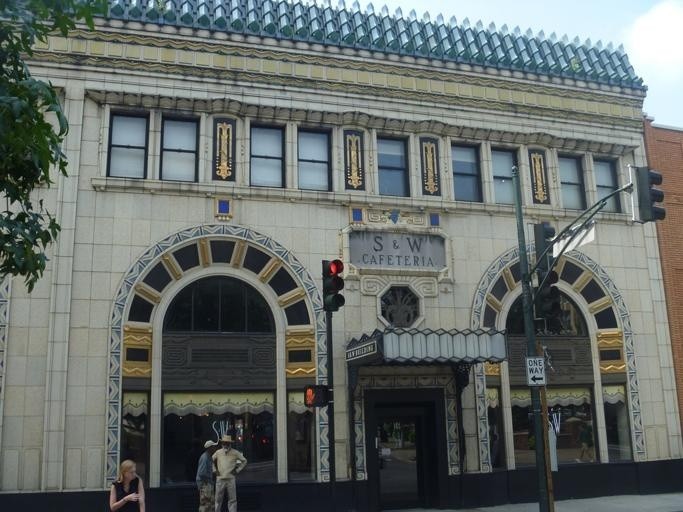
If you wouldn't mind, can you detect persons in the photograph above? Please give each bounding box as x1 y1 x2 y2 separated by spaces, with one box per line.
210 432 247 511
108 460 146 511
195 439 215 511
575 421 594 463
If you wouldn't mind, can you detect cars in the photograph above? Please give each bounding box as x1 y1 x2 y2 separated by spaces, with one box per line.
553 416 591 447
233 409 312 474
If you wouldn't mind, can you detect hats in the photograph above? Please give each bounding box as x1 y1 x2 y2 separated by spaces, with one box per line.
220 435 234 442
204 440 217 448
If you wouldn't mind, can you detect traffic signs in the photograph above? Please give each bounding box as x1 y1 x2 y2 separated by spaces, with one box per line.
524 356 547 387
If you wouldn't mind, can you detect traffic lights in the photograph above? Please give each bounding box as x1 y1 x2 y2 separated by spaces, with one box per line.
537 268 560 317
321 259 346 311
634 166 666 223
304 384 328 408
533 222 555 268
